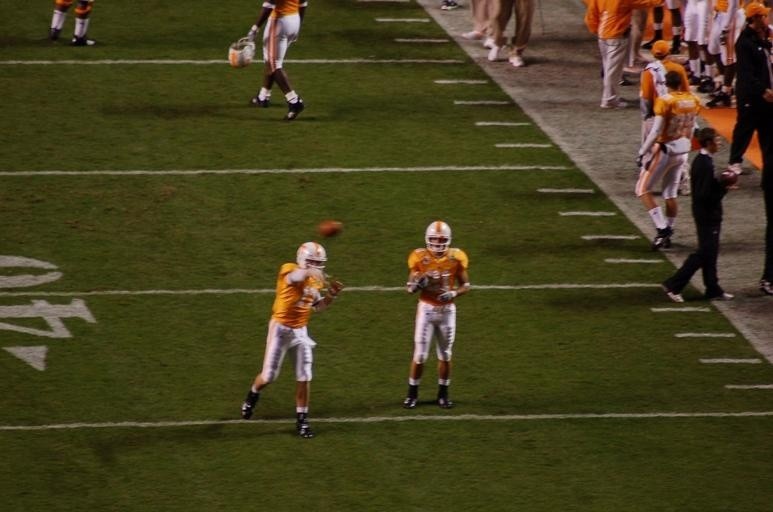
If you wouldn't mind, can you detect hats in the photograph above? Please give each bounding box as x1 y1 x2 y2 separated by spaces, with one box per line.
653 40 670 56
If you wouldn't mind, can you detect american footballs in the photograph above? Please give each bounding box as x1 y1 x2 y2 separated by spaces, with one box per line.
318 220 342 234
721 168 738 185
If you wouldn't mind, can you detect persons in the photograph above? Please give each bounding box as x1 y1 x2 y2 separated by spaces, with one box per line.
440 0 535 67
242 241 344 439
403 221 471 409
245 0 308 122
49 0 96 47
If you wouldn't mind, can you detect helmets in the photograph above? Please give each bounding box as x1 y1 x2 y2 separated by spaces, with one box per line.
425 221 451 259
229 36 256 67
296 242 328 271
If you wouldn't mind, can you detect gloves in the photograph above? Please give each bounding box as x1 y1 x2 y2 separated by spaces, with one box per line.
408 276 428 294
326 280 343 298
437 290 456 302
305 268 329 285
247 25 260 41
636 155 643 167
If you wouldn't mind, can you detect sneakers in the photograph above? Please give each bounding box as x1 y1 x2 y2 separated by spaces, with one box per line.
682 60 738 108
487 46 500 61
250 97 270 107
600 102 629 108
463 30 487 39
296 419 315 438
617 79 633 87
642 39 656 49
442 1 458 10
728 163 743 175
759 279 773 295
483 33 496 47
71 35 95 45
284 96 305 120
651 226 673 250
708 293 734 300
49 28 60 40
662 284 684 303
403 393 418 409
241 400 259 419
680 189 691 195
671 44 681 55
437 397 453 408
508 51 525 67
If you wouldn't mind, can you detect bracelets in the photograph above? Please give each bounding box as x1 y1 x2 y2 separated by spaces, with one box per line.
326 289 337 299
450 289 457 298
251 24 260 33
